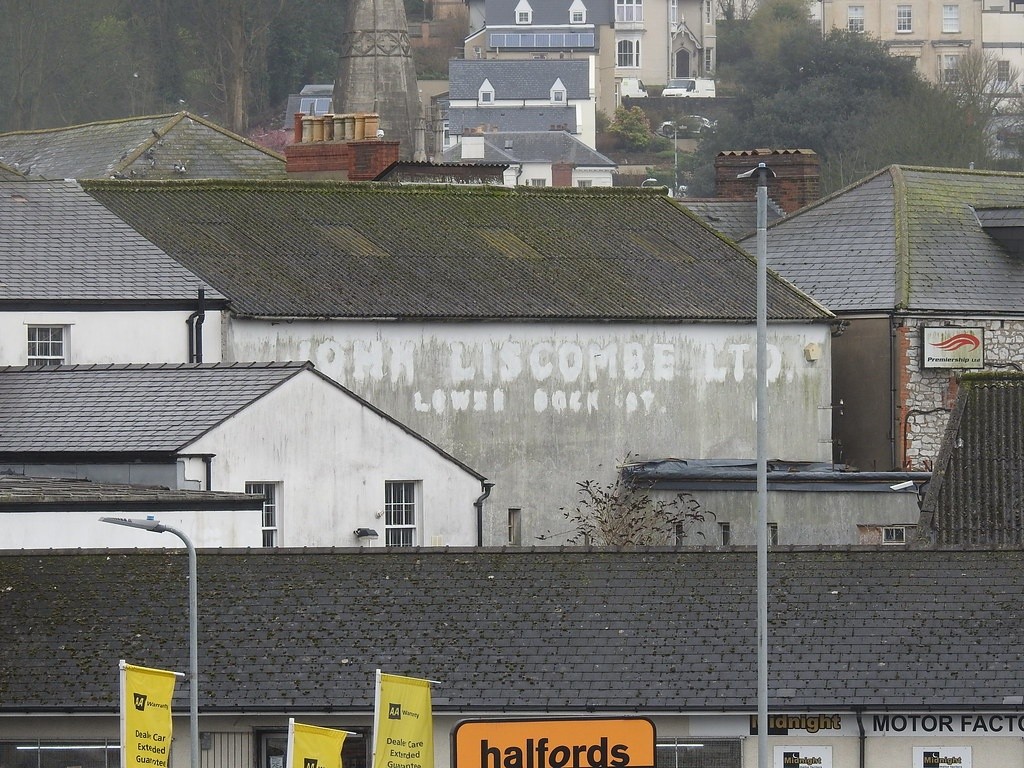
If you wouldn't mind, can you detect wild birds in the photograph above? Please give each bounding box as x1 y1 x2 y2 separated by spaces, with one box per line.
108 127 187 180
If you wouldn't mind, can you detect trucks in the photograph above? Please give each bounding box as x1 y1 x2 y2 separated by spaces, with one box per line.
616 77 649 98
659 76 715 99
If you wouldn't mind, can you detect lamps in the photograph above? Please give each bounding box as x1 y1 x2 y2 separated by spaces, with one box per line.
354 528 378 540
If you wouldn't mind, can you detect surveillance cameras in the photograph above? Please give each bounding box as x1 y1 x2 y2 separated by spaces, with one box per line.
890 481 914 491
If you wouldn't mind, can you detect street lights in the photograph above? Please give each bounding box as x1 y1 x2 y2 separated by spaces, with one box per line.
736 162 779 767
98 515 201 768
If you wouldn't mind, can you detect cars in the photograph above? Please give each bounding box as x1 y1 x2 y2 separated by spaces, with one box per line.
662 114 716 139
